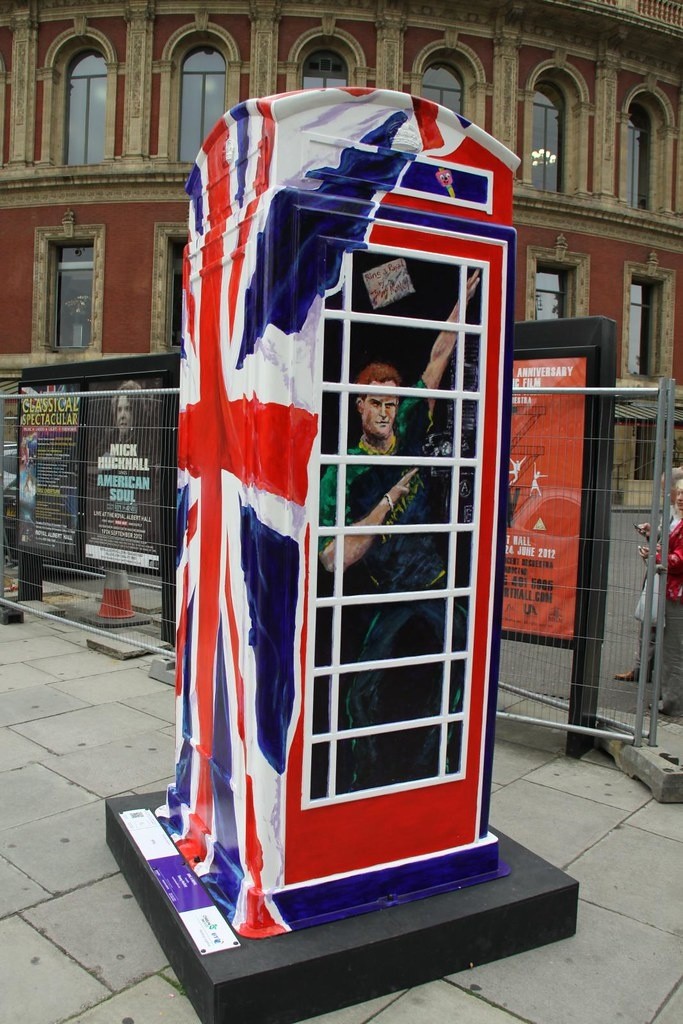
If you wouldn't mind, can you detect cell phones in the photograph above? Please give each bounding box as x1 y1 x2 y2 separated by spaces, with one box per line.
633 524 646 534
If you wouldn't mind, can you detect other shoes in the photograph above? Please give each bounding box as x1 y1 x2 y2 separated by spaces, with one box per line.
615 670 639 681
648 700 663 712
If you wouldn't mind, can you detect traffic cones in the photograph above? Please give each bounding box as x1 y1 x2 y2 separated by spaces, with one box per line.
96 567 136 618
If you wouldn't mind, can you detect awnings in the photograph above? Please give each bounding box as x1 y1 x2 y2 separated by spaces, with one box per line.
614 402 683 423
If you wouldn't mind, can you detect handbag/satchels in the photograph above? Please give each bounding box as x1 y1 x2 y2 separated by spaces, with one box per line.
633 573 666 627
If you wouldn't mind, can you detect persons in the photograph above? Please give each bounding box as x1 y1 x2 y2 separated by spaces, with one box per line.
639 480 683 718
615 468 683 683
88 378 156 457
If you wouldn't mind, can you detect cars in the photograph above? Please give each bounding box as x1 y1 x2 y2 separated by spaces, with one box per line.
3 444 19 559
